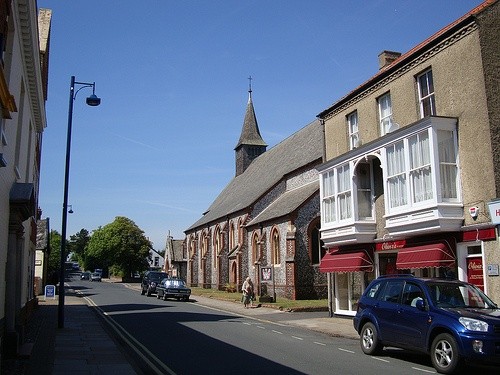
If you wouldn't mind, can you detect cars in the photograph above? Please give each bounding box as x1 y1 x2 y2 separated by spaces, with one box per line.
155 278 191 300
72 262 79 271
79 269 101 282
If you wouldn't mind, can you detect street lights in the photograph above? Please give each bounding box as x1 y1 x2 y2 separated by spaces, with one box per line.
58 75 102 330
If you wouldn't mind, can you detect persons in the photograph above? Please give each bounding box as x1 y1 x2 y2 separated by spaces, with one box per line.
241 277 255 308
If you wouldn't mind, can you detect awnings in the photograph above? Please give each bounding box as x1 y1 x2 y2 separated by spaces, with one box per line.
319 247 375 273
396 235 456 270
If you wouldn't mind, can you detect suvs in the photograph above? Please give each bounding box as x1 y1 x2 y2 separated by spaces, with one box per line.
141 271 168 297
353 273 500 373
